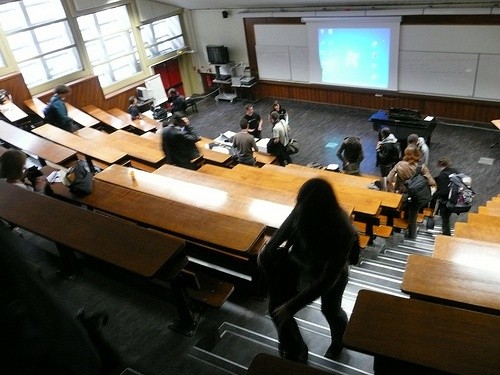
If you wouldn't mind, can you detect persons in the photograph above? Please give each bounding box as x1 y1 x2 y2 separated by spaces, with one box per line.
271 111 292 168
128 96 143 120
161 112 201 172
43 84 72 132
243 104 263 139
0 225 101 375
386 132 460 240
376 127 400 177
257 177 360 365
336 136 365 171
0 148 48 196
168 88 188 117
233 119 259 167
269 100 290 132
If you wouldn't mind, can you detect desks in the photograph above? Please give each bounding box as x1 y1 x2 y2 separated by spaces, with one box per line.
137 98 155 113
0 99 500 375
231 82 260 103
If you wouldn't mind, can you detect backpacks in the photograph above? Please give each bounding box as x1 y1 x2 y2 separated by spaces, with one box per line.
43 98 63 127
404 163 432 206
438 172 473 208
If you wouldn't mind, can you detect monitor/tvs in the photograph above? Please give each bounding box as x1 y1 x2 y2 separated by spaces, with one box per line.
207 45 229 64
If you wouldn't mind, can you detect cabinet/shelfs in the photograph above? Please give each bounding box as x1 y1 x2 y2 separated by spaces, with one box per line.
209 61 237 102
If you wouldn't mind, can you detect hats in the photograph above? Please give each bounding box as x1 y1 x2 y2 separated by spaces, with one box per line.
55 85 69 94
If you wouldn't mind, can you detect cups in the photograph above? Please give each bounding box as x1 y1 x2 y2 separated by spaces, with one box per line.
129 170 135 180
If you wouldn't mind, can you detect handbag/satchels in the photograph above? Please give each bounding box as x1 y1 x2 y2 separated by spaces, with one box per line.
153 108 167 120
286 138 299 155
66 164 93 199
257 245 302 293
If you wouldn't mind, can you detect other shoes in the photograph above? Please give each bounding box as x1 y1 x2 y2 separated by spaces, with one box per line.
323 339 344 361
405 233 414 240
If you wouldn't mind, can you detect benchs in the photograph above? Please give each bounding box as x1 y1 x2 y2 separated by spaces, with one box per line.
130 159 156 173
352 221 393 237
379 215 410 229
76 153 109 170
184 272 235 309
